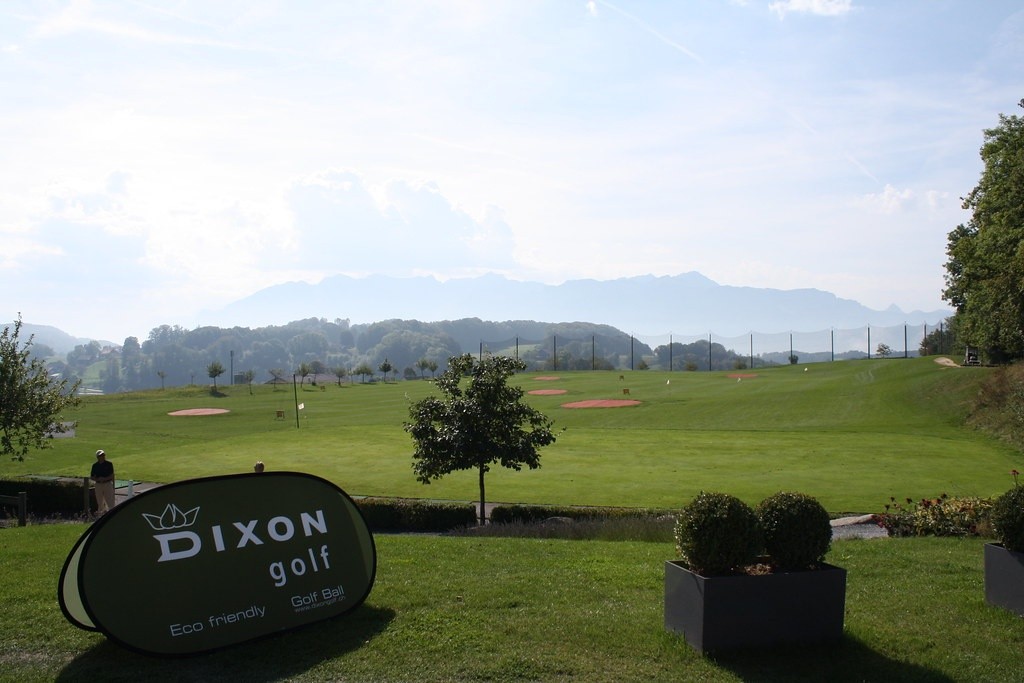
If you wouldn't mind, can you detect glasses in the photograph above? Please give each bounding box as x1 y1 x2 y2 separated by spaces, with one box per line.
97 454 105 458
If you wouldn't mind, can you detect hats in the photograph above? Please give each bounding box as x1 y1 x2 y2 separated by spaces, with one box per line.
96 450 105 457
254 463 264 470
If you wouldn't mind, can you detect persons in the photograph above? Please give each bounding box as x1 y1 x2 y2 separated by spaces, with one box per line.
253 462 264 472
91 450 115 517
